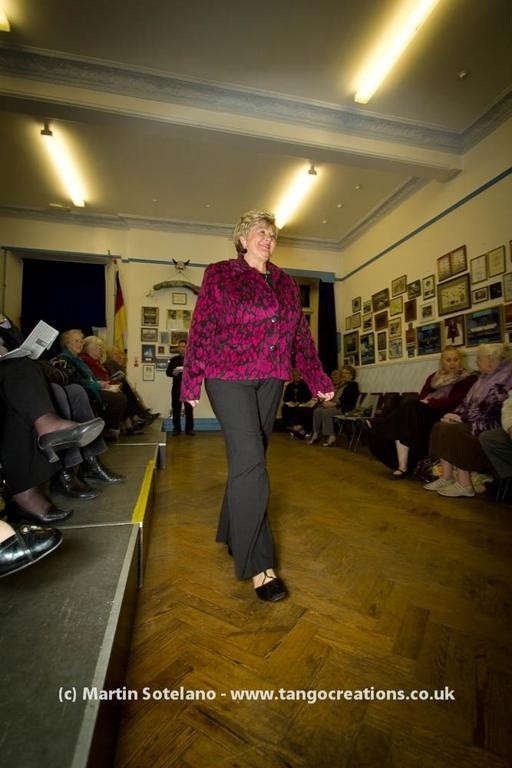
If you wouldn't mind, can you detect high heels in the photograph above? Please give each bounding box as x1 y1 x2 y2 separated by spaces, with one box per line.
35 417 105 464
6 496 74 524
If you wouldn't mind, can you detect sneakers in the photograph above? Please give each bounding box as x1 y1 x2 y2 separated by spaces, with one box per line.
305 436 321 445
421 476 457 491
322 439 338 447
435 479 477 497
387 467 410 480
250 568 290 602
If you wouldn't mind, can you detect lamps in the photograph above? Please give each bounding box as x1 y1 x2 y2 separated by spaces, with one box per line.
39 118 88 209
274 161 318 232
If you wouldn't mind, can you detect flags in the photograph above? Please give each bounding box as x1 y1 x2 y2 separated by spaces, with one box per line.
113 263 127 352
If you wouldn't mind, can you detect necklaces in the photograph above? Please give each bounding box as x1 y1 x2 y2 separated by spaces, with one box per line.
263 270 269 286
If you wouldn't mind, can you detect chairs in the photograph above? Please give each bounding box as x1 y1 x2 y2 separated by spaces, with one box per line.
331 392 421 457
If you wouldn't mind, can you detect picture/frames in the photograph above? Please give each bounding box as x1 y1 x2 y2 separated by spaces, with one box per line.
141 293 194 381
343 239 512 370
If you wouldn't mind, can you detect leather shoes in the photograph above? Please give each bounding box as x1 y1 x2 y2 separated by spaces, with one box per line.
47 469 102 502
121 409 197 438
76 455 129 486
0 522 63 579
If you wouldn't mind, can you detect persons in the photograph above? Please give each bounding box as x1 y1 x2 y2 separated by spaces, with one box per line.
166 340 195 436
180 212 335 602
479 389 512 502
281 365 358 447
424 343 512 497
361 346 478 479
